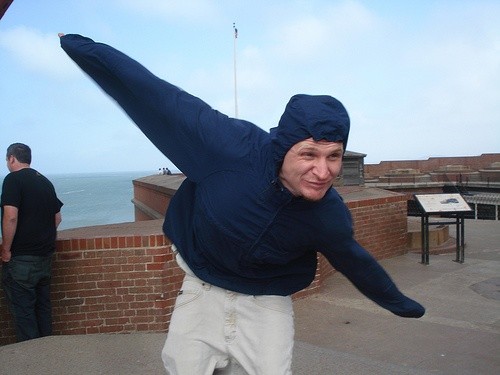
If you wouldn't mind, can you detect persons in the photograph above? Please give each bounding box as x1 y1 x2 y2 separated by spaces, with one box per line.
158 168 162 175
165 168 171 175
0 142 64 342
162 168 167 175
58 31 425 374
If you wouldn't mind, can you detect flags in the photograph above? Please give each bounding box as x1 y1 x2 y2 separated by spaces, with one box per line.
233 27 238 39
